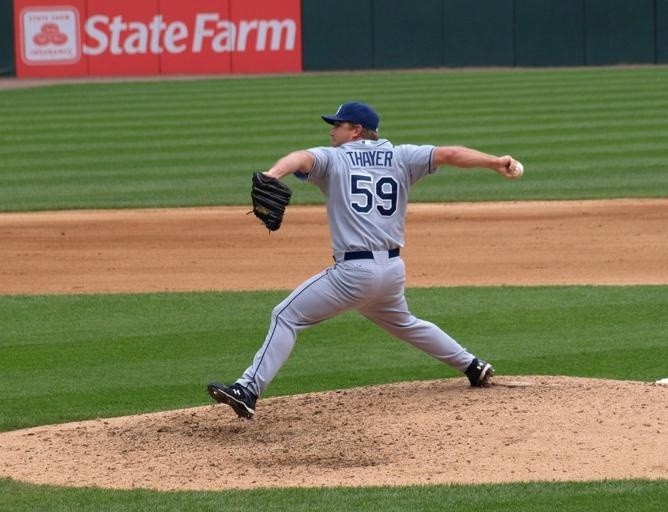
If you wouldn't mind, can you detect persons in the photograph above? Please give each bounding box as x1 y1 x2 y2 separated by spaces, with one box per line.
208 99 523 419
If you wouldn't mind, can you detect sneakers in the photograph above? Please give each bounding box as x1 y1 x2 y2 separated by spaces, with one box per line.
465 356 496 388
204 381 258 420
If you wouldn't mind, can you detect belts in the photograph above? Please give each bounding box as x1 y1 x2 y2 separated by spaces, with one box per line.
332 248 401 262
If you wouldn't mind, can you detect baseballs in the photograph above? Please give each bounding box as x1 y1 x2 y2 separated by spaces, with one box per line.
510 161 524 180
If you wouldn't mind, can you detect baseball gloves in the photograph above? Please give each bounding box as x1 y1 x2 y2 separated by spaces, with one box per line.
245 172 292 235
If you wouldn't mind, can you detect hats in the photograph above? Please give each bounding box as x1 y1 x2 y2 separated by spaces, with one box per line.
321 100 382 132
